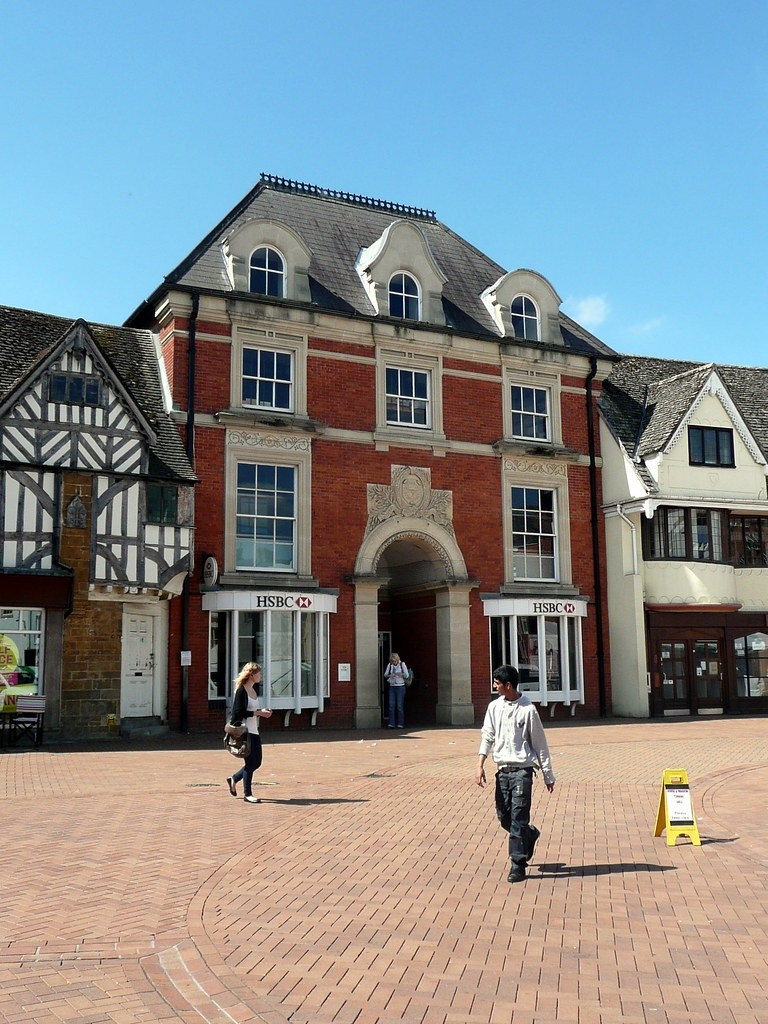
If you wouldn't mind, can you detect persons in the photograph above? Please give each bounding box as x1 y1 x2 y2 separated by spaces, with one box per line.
475 664 556 882
384 652 411 731
222 661 273 803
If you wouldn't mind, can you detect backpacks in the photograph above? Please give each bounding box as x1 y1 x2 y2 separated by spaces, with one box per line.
401 661 413 687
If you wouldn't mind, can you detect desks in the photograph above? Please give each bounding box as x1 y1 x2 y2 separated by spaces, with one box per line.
1 712 44 748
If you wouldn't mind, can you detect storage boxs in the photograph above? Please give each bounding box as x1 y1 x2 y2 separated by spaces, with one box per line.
3 695 45 713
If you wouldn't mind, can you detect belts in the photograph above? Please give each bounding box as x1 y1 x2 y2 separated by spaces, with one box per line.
502 767 520 773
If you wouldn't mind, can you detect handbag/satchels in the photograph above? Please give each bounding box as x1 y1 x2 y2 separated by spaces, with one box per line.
223 720 251 758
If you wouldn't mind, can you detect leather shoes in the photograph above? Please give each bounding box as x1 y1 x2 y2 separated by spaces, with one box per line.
507 859 526 882
526 825 540 861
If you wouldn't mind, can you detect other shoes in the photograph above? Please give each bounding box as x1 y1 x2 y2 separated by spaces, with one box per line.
388 724 404 729
244 795 261 803
227 776 237 796
